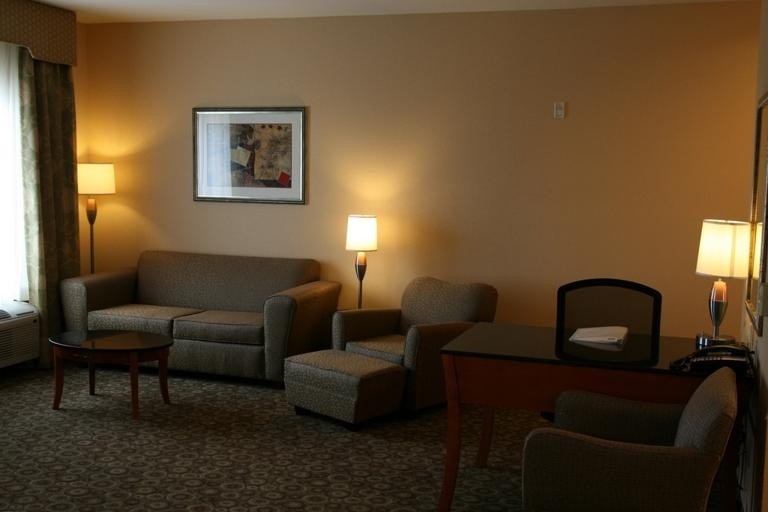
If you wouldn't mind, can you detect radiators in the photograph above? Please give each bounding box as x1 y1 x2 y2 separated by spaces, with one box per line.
0 312 39 368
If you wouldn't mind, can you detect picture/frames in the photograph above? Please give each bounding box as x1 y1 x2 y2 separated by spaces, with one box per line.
192 106 306 203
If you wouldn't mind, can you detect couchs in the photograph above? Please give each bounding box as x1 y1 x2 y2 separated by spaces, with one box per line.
522 367 738 512
59 250 341 424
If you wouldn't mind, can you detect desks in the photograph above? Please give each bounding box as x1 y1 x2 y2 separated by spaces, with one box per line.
437 322 760 512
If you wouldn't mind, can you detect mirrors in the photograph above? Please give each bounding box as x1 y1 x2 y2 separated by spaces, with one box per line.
744 88 768 337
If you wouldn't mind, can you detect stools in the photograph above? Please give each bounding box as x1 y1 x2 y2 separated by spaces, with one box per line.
285 349 407 433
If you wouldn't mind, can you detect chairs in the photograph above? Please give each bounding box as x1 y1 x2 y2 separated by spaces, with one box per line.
540 277 659 425
331 277 498 414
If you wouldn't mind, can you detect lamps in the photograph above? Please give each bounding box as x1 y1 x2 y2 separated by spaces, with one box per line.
696 219 755 350
76 164 118 275
345 214 379 309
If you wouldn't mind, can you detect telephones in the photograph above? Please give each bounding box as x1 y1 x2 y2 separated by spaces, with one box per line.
675 343 749 372
